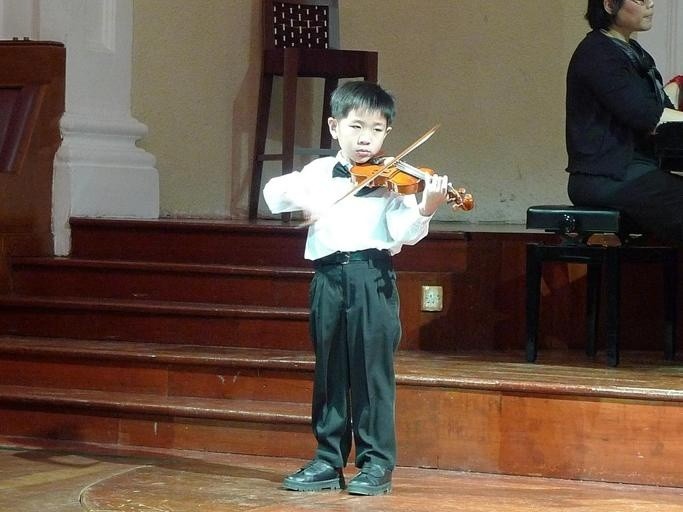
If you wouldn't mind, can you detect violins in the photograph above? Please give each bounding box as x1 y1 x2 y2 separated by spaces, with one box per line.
349 154 475 212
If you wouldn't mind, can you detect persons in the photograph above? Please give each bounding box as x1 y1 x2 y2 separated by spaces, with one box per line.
260 79 449 496
564 0 683 249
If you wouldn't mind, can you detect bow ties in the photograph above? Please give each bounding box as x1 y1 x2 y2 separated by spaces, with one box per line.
333 161 351 178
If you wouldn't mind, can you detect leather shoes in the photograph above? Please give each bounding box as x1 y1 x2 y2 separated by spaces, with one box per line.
348 465 392 496
283 459 345 492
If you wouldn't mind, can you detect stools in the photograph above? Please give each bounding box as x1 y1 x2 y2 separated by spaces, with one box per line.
518 203 679 370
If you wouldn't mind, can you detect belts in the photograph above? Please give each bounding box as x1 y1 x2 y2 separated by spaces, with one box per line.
314 250 388 265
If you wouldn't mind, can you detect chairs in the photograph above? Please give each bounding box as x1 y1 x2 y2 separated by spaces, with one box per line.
246 0 378 224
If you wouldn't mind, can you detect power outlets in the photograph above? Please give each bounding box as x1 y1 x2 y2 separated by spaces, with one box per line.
419 284 443 312
